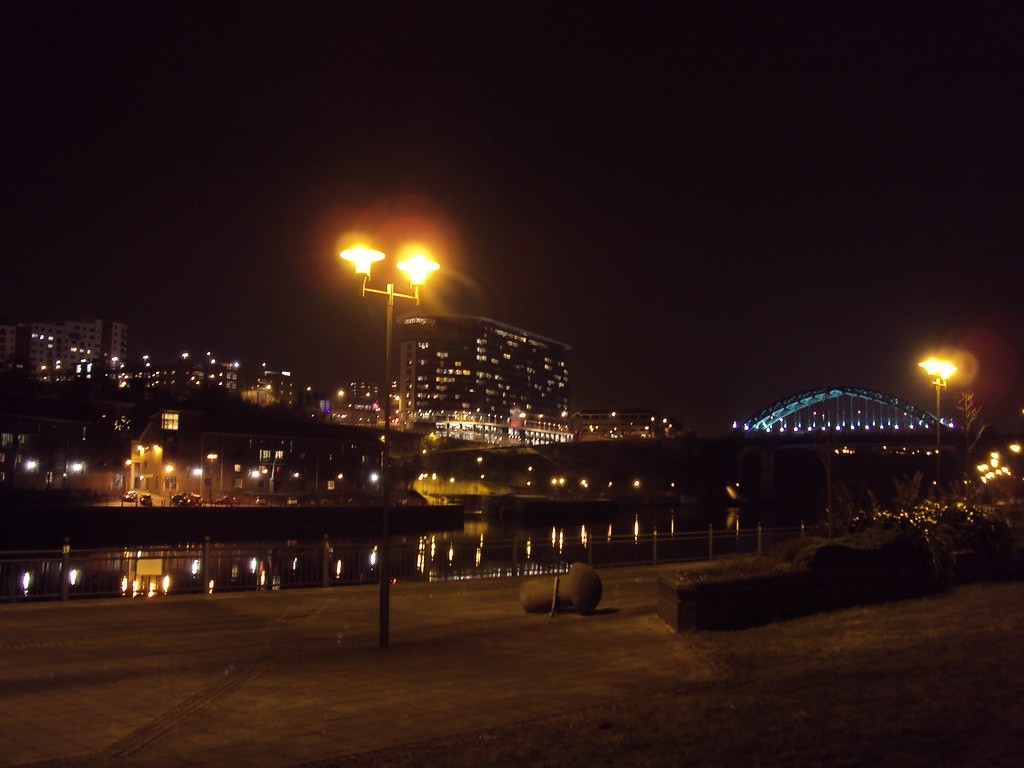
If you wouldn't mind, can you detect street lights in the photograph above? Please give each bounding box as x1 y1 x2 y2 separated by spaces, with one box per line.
207 453 218 505
338 241 441 650
919 354 959 504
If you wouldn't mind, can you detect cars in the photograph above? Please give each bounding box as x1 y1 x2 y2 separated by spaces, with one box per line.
140 493 152 506
287 496 298 507
124 490 138 502
171 491 204 506
215 495 236 506
